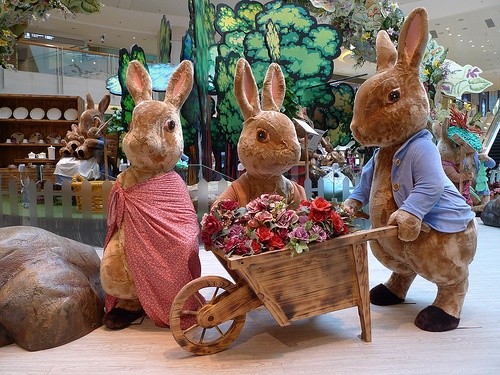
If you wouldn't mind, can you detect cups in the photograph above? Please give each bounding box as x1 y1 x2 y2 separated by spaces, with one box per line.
37 152 46 158
28 152 35 158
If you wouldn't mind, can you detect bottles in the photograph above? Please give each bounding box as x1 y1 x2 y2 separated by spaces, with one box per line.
48 145 55 160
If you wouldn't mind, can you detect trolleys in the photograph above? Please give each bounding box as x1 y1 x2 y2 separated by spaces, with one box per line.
170 207 431 356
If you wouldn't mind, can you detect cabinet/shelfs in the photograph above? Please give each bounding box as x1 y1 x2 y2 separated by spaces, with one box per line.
0 94 84 191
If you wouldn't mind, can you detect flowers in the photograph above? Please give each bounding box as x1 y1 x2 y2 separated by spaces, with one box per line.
198 192 353 254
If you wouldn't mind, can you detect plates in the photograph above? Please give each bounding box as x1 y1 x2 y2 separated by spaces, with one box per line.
46 133 61 144
0 107 12 119
29 133 43 144
13 107 28 119
30 108 46 119
10 132 24 144
64 109 78 120
47 108 62 120
